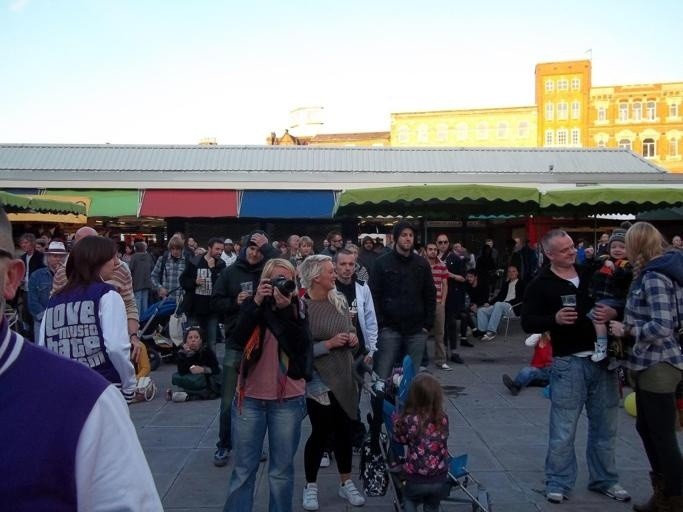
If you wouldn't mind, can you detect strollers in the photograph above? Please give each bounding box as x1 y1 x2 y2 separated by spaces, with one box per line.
349 354 496 511
139 289 180 370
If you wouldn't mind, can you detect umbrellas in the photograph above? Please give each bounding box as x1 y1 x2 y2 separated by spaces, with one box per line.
540 187 683 256
331 186 541 247
0 190 87 215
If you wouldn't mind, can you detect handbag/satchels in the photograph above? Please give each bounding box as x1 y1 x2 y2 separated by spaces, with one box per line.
169 312 187 346
136 377 155 402
172 371 208 391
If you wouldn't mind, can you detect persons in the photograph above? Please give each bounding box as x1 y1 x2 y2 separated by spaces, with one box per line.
671 236 682 248
610 223 682 511
583 221 635 370
1 204 556 512
520 227 631 505
575 232 610 261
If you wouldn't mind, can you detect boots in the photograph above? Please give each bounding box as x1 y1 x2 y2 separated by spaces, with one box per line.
633 471 683 511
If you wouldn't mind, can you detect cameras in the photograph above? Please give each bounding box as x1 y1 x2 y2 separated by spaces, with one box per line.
268 274 296 297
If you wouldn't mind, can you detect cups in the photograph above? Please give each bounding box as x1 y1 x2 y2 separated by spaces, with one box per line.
240 281 254 296
560 294 577 322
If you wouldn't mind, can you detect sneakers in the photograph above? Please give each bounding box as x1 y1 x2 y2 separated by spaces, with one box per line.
546 493 565 504
172 391 188 402
600 484 631 502
503 374 520 396
213 447 365 510
591 343 608 363
435 329 497 371
607 357 623 371
362 430 389 497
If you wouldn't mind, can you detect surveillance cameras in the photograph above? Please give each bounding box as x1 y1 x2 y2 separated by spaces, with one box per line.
549 165 553 171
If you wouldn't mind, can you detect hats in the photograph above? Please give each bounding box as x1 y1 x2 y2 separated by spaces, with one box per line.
607 221 632 254
392 219 416 240
43 241 70 255
246 231 270 247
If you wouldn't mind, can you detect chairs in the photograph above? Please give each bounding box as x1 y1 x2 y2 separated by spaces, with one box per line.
503 303 521 342
